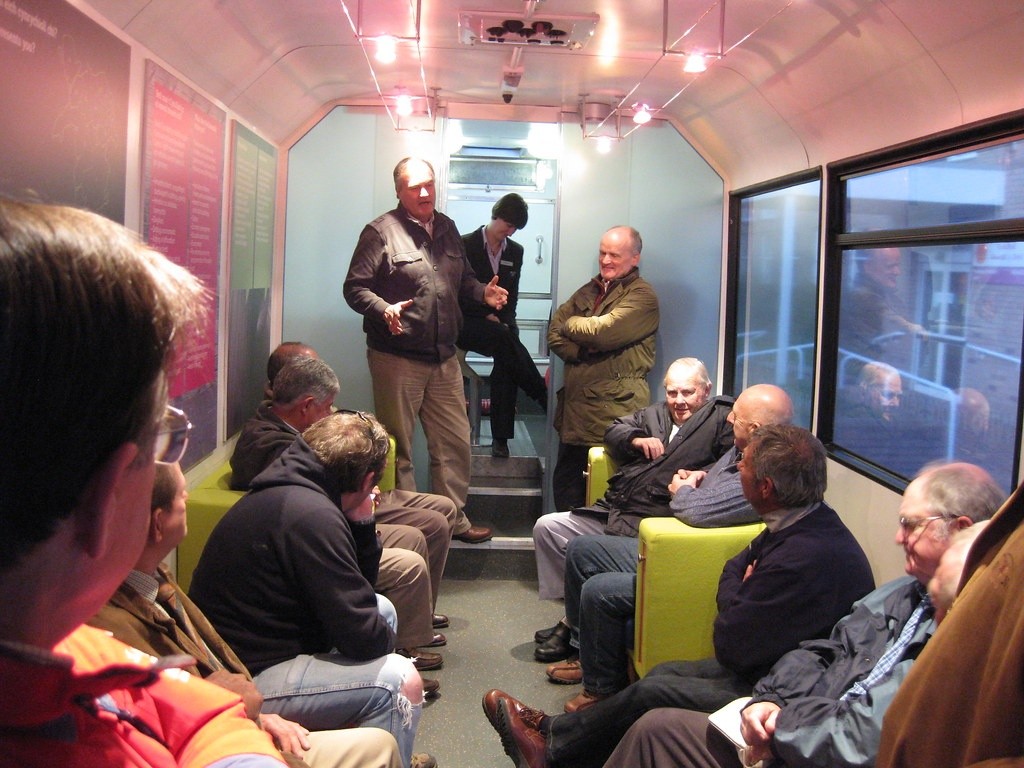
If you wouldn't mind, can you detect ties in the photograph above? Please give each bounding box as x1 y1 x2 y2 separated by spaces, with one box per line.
156 577 225 671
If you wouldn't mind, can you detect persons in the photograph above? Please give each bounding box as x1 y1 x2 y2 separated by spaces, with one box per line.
548 225 660 512
604 459 1023 767
835 246 996 491
545 383 794 714
482 423 876 768
187 407 437 768
84 462 405 768
262 340 457 646
0 198 285 768
458 193 548 458
343 157 493 544
228 353 445 698
533 356 736 663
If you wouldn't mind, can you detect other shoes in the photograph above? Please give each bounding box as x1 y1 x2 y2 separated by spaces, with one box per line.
491 439 509 458
538 380 549 415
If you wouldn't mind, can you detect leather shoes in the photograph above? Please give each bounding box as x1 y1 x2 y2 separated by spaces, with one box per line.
563 688 604 713
482 688 546 734
534 627 554 643
395 647 444 670
496 696 551 768
432 613 450 628
425 633 446 647
450 525 493 544
546 659 584 684
533 615 577 662
422 678 440 698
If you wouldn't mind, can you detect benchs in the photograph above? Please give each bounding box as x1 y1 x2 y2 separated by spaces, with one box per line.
176 434 396 597
583 446 767 680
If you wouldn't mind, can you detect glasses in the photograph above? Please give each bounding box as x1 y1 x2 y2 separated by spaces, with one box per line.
334 409 376 444
90 406 195 465
900 514 976 537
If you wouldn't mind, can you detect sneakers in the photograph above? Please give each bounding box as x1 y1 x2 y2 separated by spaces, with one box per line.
409 752 437 768
